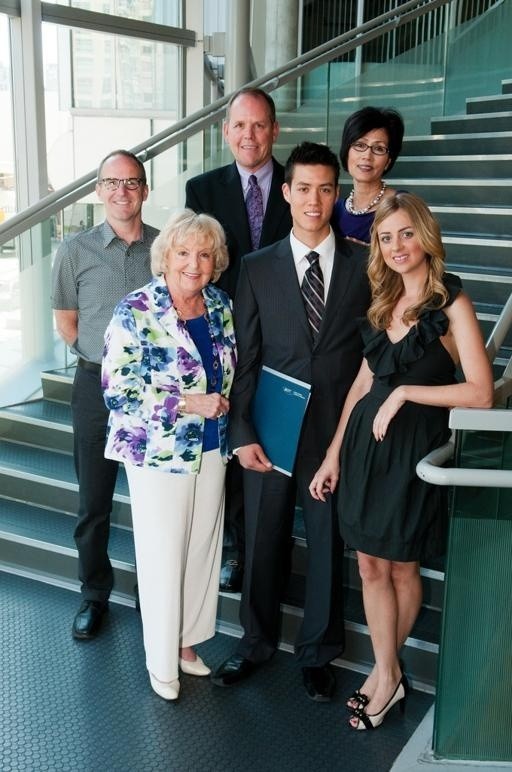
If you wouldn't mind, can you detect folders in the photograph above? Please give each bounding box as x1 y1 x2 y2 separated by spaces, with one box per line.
249 365 313 479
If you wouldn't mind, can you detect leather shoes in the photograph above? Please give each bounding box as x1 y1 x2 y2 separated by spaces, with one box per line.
220 559 243 593
149 673 180 701
212 653 256 687
303 665 340 703
178 654 212 675
72 600 108 640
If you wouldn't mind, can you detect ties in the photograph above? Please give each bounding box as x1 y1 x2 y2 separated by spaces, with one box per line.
300 251 324 347
245 175 264 250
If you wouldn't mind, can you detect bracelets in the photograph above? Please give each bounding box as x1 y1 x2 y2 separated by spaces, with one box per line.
176 394 186 412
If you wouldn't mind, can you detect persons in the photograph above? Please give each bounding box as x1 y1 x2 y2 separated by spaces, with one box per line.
210 142 375 705
309 190 493 731
331 107 405 244
50 151 163 640
184 90 294 595
101 208 239 700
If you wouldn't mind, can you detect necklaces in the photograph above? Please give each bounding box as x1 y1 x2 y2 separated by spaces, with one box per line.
345 180 386 215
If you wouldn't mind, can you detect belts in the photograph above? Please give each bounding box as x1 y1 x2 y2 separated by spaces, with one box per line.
78 357 101 374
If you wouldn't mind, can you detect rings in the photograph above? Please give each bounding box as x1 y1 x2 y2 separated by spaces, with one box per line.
218 412 224 419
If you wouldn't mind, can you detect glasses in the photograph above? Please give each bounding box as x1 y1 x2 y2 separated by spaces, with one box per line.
352 141 389 155
98 178 144 191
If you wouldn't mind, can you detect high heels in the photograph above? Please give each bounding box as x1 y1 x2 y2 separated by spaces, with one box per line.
348 675 410 730
347 659 404 711
